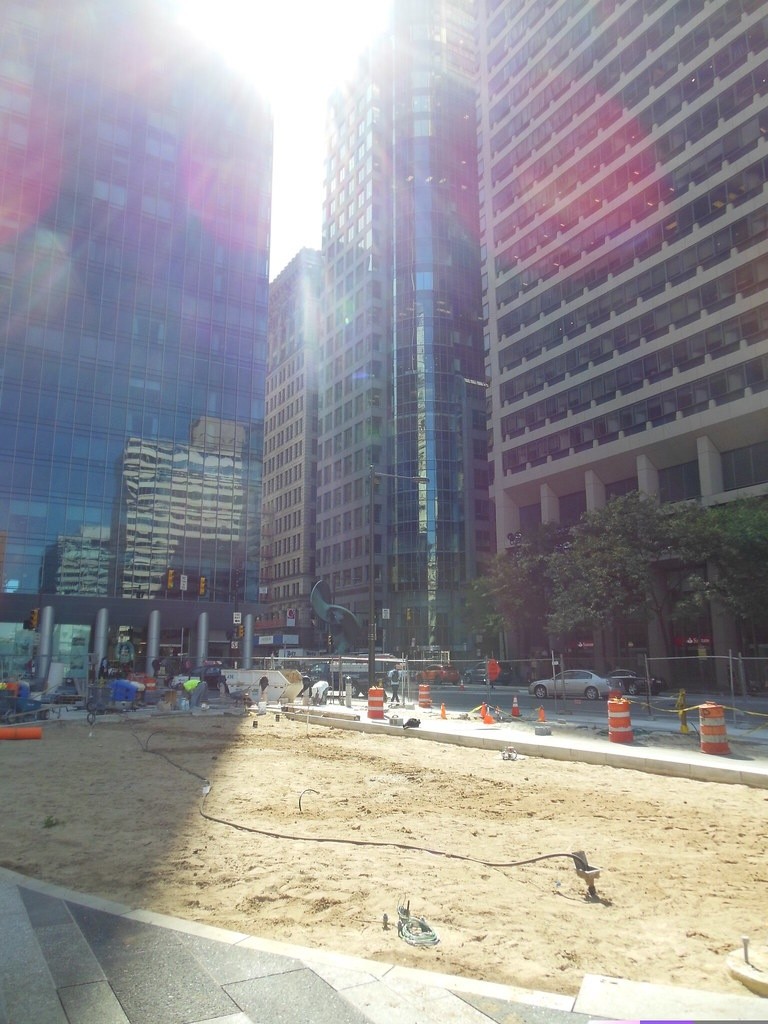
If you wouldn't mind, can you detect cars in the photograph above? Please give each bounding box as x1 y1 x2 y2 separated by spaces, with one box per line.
163 660 234 691
465 660 514 686
603 667 668 695
414 662 466 686
527 668 626 701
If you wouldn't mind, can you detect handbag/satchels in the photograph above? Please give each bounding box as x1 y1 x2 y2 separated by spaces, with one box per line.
403 718 421 730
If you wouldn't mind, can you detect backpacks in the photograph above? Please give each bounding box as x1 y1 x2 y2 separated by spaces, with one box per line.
392 670 400 682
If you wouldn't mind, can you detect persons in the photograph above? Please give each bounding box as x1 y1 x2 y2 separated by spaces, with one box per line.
388 665 400 706
101 656 108 679
152 658 160 678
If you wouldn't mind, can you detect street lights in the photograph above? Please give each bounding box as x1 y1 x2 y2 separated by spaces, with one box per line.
369 463 429 690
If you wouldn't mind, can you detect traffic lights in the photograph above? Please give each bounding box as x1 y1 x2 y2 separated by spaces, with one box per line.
30 608 38 626
199 576 207 596
328 635 333 645
238 625 245 638
168 569 175 589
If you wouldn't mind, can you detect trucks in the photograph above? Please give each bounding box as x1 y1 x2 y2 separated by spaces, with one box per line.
304 652 400 700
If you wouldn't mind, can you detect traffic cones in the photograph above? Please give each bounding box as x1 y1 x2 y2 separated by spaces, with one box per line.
456 679 467 693
483 706 495 724
509 693 523 718
479 701 487 718
535 705 549 723
440 702 449 720
